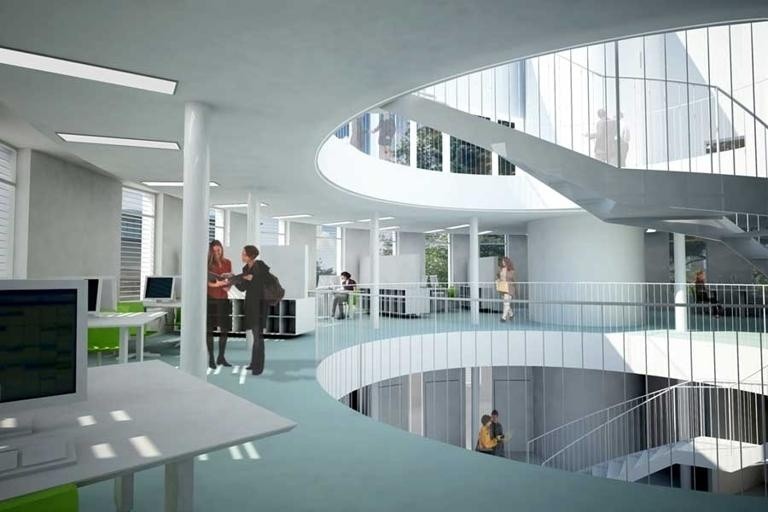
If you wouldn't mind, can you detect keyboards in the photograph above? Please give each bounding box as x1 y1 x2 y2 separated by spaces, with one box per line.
0 433 79 479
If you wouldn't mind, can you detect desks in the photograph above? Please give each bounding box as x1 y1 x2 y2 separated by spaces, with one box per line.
707 288 759 318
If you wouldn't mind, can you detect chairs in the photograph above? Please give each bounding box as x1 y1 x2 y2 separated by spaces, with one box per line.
343 286 360 320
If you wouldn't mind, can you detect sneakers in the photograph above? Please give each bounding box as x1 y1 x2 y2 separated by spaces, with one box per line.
336 314 346 319
209 359 262 375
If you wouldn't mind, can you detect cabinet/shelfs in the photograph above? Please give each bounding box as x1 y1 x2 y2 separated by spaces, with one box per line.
359 287 430 319
459 286 493 312
174 297 316 339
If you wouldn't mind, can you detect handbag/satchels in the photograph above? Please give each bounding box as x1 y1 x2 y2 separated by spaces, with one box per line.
495 280 509 293
262 273 284 307
475 448 497 456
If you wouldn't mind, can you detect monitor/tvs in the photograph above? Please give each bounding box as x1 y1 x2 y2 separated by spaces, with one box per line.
318 275 342 289
100 276 117 311
82 277 103 315
141 275 176 303
0 278 89 438
169 276 181 299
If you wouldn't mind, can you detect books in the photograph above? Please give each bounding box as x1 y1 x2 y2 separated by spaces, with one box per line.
208 270 244 288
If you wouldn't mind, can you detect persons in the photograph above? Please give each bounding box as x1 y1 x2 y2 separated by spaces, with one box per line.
206 239 232 368
614 111 627 167
478 415 502 455
709 291 717 315
693 272 708 314
490 410 505 456
496 255 518 322
221 244 272 375
370 111 396 161
331 271 356 320
587 108 616 165
